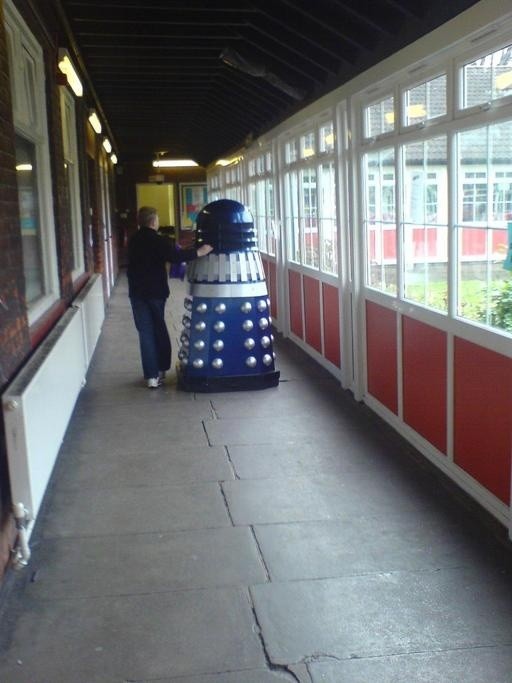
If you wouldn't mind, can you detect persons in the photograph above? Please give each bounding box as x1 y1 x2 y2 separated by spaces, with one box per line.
128 207 213 389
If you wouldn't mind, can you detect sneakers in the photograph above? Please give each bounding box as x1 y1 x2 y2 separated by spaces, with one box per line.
147 371 165 388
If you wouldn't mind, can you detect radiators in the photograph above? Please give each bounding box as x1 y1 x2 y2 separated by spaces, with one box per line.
1 273 107 570
